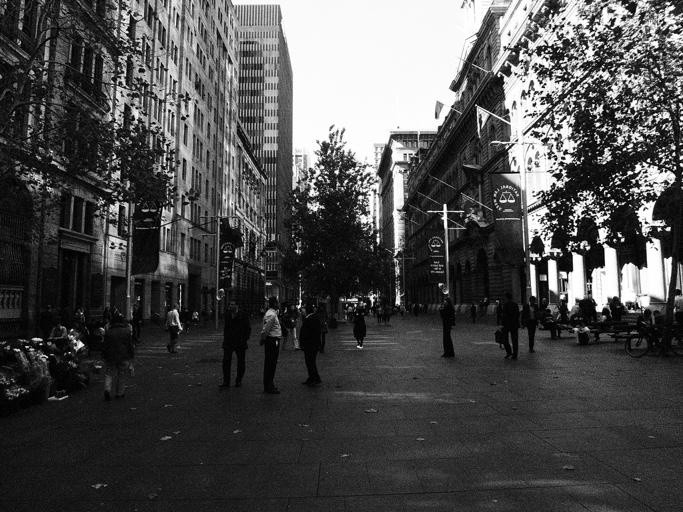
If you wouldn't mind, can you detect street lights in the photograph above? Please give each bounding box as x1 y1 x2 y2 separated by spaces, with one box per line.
197 214 238 331
425 204 465 295
489 132 552 302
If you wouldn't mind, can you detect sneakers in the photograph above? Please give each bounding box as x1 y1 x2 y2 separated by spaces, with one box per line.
264 388 280 394
217 382 230 387
104 390 112 402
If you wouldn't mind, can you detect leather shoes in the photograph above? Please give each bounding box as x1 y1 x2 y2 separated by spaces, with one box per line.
505 353 512 358
314 379 322 384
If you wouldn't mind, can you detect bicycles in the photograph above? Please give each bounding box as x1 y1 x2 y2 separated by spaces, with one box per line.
624 316 682 359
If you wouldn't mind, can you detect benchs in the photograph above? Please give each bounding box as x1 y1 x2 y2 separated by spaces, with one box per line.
537 309 646 349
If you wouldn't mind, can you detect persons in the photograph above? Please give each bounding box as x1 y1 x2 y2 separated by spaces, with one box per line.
439 298 456 358
261 297 282 394
470 292 682 360
345 295 389 349
394 302 428 317
218 299 252 388
165 303 183 353
45 302 141 400
275 298 328 386
191 309 199 321
